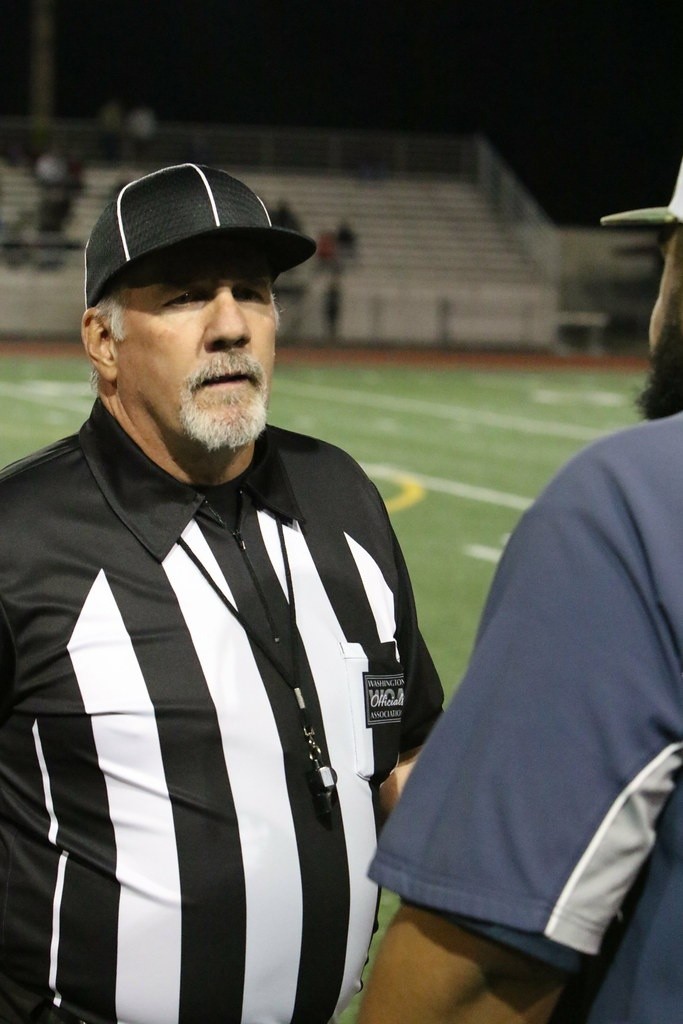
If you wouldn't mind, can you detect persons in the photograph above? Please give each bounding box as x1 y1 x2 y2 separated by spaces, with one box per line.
0 162 444 1024
357 154 683 1024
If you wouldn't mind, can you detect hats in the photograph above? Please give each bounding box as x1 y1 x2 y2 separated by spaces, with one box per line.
600 160 683 223
84 163 317 309
